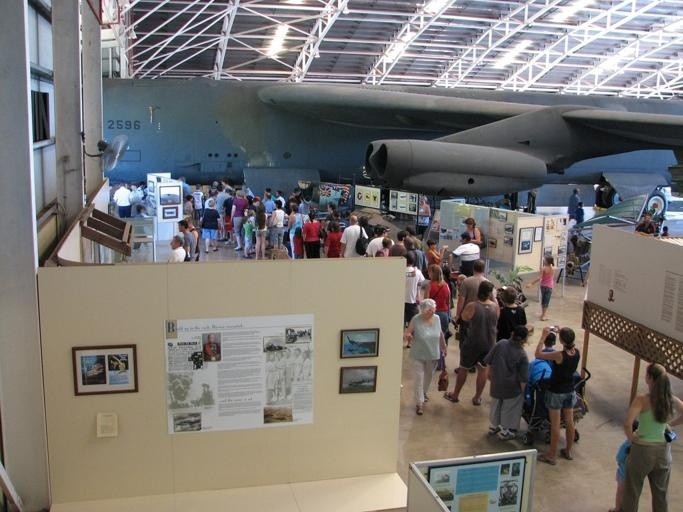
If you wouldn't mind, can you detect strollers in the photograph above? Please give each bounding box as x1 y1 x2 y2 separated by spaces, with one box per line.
522 359 590 446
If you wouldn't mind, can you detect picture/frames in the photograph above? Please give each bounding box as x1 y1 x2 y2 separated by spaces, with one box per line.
340 366 377 393
428 457 526 512
72 345 138 396
518 227 534 254
341 329 379 358
534 227 543 242
148 180 181 219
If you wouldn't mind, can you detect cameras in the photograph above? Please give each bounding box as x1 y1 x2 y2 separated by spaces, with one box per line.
550 326 559 332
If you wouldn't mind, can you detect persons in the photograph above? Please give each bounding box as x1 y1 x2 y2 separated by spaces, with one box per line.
203 333 221 362
567 189 584 224
607 418 640 512
482 323 529 443
403 297 446 416
533 324 581 467
619 361 683 512
505 190 537 214
442 280 500 406
264 347 311 404
404 215 559 390
635 214 668 236
109 173 409 266
594 181 609 214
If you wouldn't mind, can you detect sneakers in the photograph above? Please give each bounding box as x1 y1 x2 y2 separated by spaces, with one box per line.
488 427 517 440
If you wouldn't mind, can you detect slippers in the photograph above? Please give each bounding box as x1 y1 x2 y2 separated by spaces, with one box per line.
472 397 481 405
444 392 458 402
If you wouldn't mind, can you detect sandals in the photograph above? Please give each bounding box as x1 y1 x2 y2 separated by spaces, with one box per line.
416 406 423 415
561 448 574 459
537 454 556 465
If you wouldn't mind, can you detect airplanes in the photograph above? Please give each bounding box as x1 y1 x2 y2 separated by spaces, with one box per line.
104 79 683 198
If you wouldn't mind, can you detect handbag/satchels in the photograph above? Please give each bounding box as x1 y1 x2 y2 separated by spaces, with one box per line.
438 372 448 390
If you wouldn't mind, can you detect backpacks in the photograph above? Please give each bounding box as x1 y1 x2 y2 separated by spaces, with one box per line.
355 226 368 255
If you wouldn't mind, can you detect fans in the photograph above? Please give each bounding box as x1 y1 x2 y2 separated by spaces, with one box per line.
83 134 130 171
